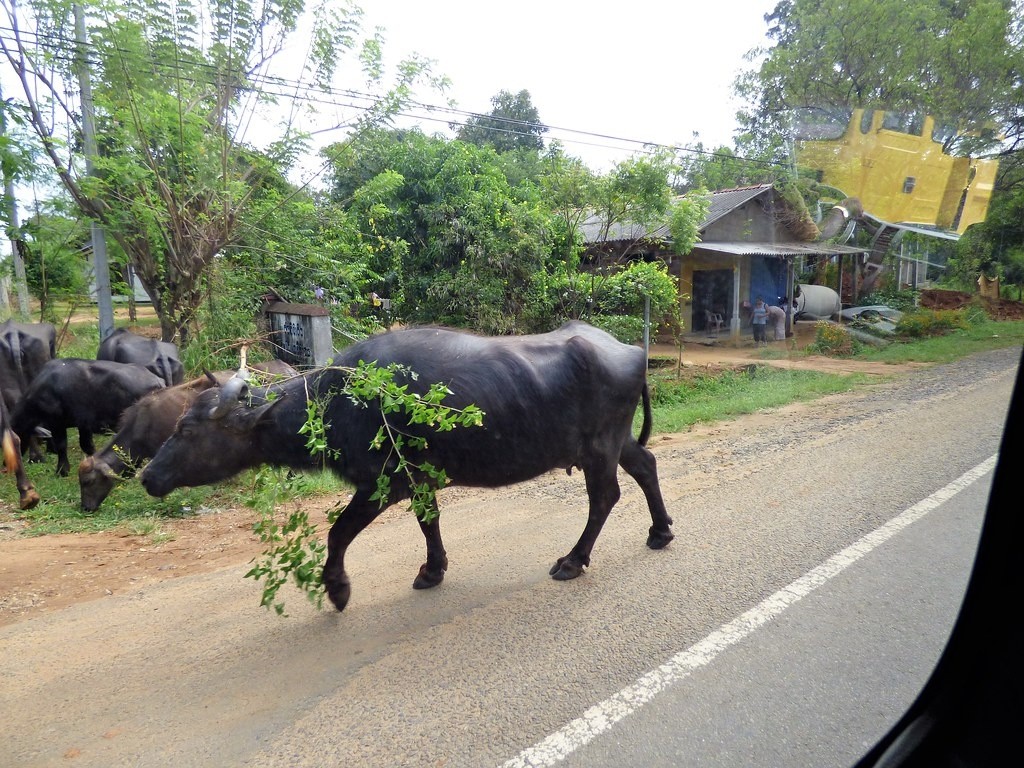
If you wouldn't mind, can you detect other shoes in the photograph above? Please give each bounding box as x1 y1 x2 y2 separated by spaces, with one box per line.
763 342 767 347
754 342 758 347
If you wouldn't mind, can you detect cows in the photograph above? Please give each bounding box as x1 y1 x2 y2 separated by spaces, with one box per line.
0 320 676 613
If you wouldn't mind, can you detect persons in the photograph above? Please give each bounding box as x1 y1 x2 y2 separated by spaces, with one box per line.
748 296 770 348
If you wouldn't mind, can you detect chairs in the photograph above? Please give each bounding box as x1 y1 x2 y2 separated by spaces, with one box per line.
704 309 725 337
